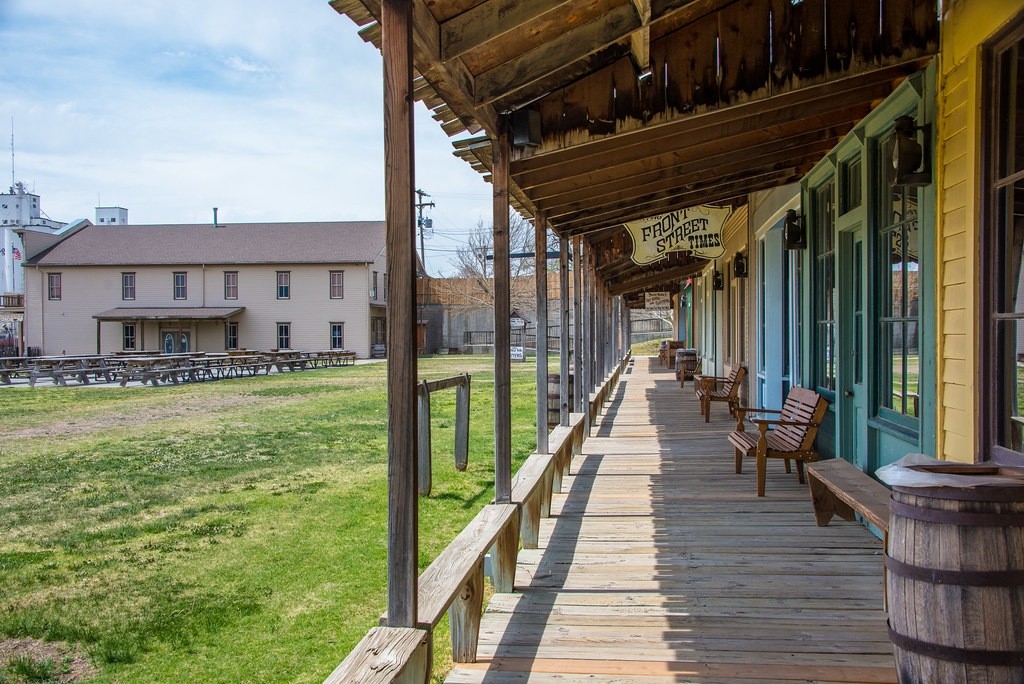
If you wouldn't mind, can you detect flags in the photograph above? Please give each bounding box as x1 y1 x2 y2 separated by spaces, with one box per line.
13 247 21 260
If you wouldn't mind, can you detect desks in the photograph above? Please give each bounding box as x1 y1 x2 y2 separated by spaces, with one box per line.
0 357 32 379
17 350 358 387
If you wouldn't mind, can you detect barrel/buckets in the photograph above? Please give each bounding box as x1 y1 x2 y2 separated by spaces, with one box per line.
661 340 670 356
547 374 575 428
886 466 1024 684
675 349 697 381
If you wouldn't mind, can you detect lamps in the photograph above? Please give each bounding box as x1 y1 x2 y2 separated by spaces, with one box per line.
783 209 806 250
734 252 748 277
713 271 723 290
680 296 687 307
892 116 932 187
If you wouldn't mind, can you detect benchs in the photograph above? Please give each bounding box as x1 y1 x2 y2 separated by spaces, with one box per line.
804 457 894 613
694 375 715 393
0 354 358 387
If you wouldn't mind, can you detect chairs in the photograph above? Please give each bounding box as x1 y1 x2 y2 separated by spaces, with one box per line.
695 363 746 423
727 386 829 496
680 357 702 388
